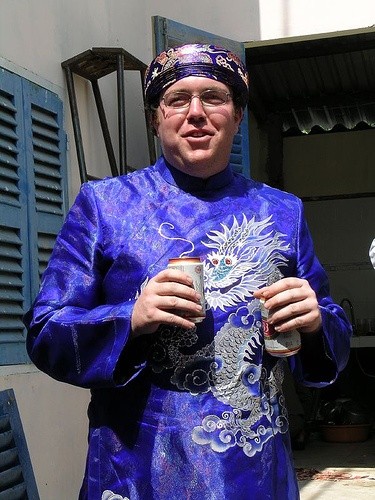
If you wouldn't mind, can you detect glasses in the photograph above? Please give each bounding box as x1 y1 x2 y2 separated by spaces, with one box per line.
160 90 234 109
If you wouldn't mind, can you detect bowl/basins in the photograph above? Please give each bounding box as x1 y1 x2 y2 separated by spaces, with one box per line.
320 423 370 442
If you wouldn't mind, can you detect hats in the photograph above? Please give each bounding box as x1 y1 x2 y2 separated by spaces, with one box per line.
144 42 248 114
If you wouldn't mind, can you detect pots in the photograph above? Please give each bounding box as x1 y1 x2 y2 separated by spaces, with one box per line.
315 398 372 424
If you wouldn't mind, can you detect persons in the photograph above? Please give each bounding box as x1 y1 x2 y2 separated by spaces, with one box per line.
20 39 355 500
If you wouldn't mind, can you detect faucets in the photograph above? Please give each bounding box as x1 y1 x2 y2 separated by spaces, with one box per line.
339 298 358 336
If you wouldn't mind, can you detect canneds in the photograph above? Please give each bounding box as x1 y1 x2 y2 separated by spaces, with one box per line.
260 292 302 357
167 257 206 322
